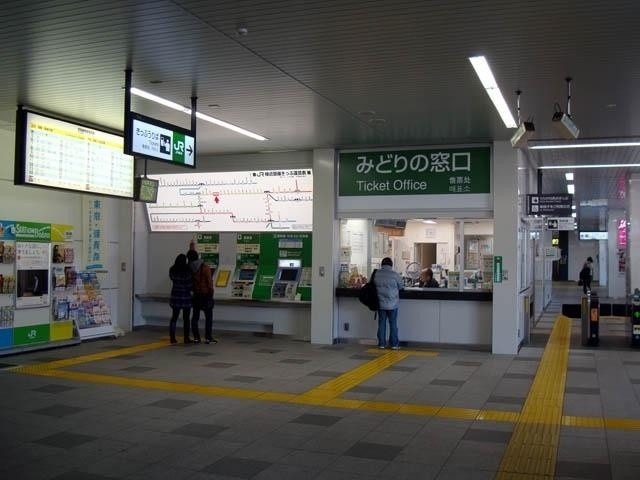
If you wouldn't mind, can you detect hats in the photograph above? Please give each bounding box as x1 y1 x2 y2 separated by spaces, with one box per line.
381 257 392 266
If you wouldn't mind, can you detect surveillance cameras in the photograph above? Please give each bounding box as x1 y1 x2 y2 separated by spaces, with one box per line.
510 122 536 149
551 109 581 140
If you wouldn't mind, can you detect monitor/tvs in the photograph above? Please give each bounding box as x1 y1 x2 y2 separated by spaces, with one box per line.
237 266 258 282
276 267 301 284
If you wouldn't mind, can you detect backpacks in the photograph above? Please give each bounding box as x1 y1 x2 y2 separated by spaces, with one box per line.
579 262 591 281
358 269 380 310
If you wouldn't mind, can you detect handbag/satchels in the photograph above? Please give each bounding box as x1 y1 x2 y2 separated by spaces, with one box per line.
193 293 214 309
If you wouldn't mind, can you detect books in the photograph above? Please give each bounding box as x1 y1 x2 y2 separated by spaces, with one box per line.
53 267 111 327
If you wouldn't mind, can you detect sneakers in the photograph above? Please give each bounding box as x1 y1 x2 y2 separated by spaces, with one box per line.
379 346 385 349
194 338 201 344
391 346 400 350
205 338 217 344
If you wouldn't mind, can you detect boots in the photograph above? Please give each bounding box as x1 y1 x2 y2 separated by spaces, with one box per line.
184 332 194 344
170 331 178 343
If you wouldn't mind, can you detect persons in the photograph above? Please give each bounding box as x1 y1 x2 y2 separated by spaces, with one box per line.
373 257 403 350
187 249 217 343
587 256 593 283
32 273 42 296
169 254 195 344
419 268 439 288
580 264 590 294
